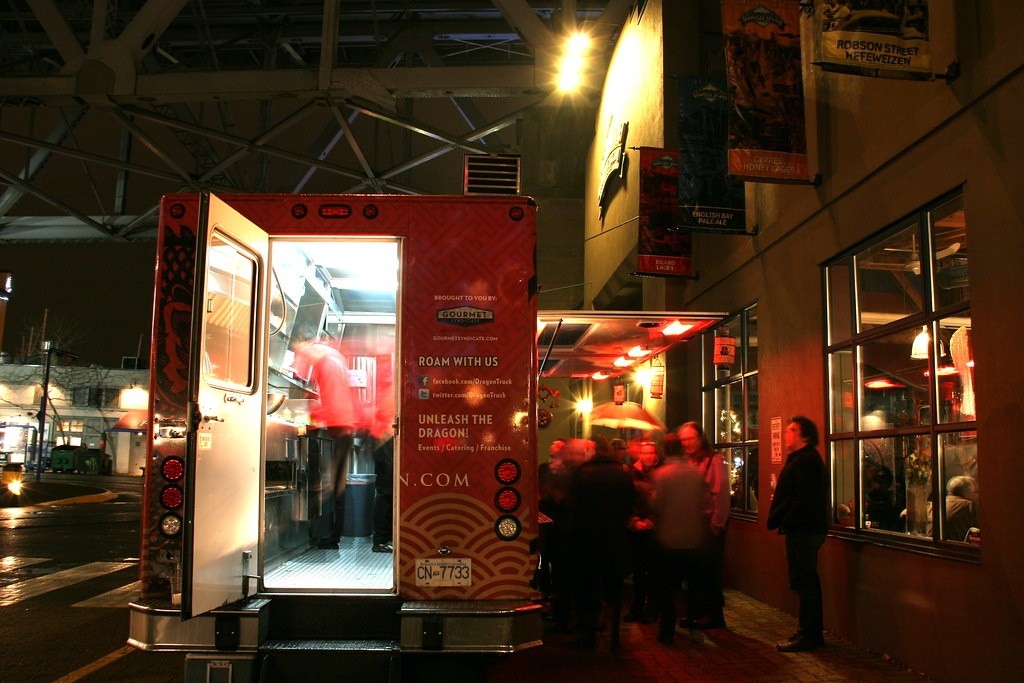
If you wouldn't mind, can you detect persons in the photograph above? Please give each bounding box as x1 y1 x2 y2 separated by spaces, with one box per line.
289 337 398 552
765 414 831 652
538 421 732 652
943 475 976 543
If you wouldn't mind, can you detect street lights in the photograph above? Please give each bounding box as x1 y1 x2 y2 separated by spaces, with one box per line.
32 339 55 485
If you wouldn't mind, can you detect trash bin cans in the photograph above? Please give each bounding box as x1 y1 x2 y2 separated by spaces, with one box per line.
341 473 377 538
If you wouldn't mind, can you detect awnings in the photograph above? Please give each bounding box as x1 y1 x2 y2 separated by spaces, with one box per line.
537 310 729 379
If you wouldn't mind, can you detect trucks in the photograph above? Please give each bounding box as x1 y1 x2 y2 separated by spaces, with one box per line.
126 190 730 683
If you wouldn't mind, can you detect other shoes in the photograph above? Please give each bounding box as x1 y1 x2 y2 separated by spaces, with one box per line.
679 609 725 629
624 611 658 624
657 633 674 645
777 630 824 651
689 628 703 642
